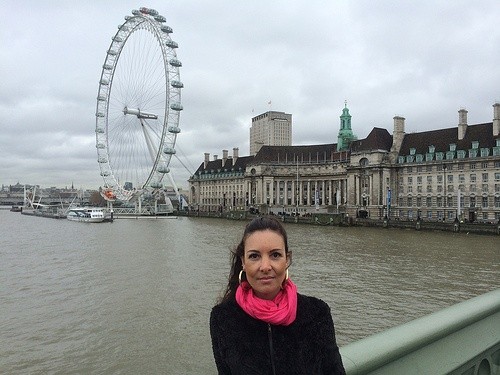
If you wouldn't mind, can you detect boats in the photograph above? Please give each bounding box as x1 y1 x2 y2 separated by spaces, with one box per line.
67 207 104 224
7 204 21 212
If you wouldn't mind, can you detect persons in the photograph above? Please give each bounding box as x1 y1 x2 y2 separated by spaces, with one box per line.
210 217 346 375
